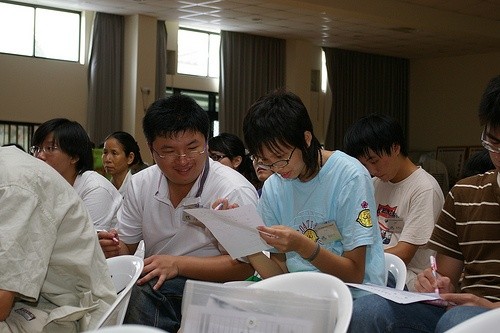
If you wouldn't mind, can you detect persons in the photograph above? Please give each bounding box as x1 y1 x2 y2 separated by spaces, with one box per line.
0 146 118 333
211 88 388 300
95 93 260 333
457 152 497 181
350 74 500 333
345 113 446 291
3 132 273 195
29 118 124 232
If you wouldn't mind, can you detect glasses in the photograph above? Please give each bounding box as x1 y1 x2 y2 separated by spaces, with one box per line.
209 154 228 162
152 145 207 160
480 123 500 154
249 154 258 161
256 145 298 171
29 145 62 154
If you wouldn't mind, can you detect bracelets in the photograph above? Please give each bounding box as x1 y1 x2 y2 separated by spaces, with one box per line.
303 241 320 262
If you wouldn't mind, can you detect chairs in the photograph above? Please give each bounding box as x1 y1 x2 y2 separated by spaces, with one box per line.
84 252 408 333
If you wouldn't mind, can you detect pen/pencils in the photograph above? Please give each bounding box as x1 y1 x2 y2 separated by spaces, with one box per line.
102 229 119 243
214 187 239 210
429 255 440 298
